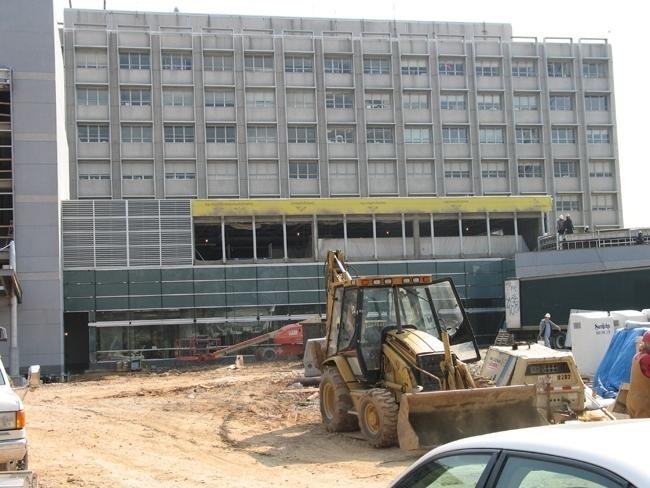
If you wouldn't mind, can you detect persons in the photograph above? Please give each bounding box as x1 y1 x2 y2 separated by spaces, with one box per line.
556 213 565 232
635 230 644 241
538 311 561 348
562 214 575 234
585 225 592 232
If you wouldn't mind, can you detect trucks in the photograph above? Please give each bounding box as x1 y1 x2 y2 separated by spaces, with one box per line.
498 262 650 349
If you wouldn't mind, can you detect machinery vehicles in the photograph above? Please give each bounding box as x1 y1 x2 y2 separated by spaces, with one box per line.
169 310 327 367
90 330 261 362
294 248 557 455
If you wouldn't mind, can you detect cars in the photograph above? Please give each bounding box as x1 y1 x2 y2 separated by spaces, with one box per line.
0 352 41 487
386 410 650 487
